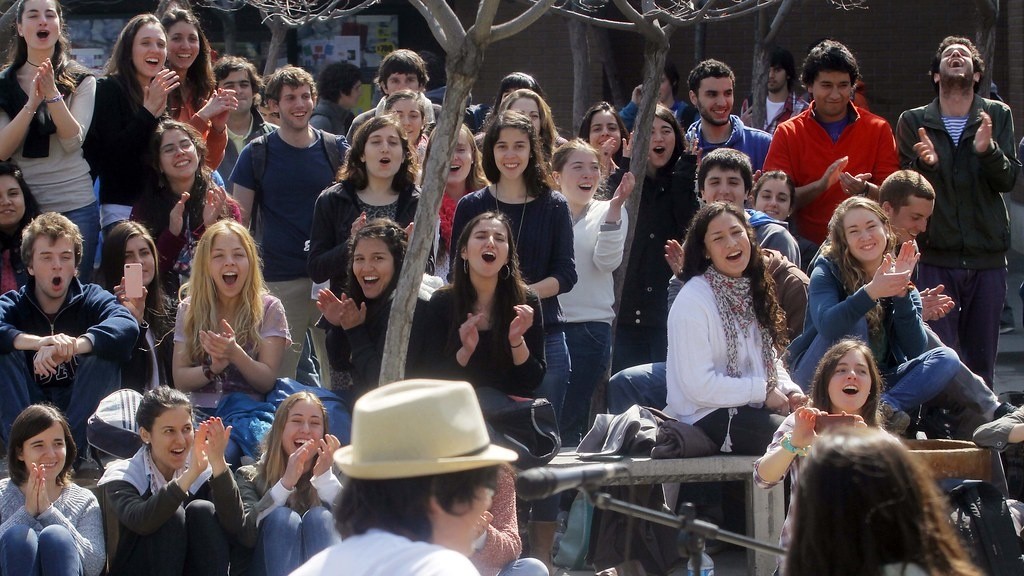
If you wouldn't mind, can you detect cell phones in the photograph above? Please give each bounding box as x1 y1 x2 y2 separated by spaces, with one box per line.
814 413 856 437
124 263 143 298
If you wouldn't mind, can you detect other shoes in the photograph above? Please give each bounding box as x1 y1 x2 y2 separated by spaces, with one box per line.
886 411 910 435
994 402 1019 420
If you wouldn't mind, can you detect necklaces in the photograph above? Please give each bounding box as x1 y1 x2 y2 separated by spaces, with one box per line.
494 182 528 251
26 60 41 68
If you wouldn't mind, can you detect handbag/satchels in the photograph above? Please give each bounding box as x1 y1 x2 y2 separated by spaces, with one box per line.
86 388 144 459
471 386 563 469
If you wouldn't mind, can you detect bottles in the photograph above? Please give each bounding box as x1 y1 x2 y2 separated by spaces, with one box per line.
552 518 566 555
687 540 715 576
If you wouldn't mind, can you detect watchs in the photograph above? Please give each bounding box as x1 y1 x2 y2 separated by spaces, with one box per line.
605 217 623 226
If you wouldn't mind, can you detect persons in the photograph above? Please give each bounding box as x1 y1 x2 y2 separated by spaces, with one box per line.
0 1 99 287
0 0 1024 575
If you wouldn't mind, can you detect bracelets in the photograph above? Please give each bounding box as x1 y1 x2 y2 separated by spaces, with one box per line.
859 183 869 198
511 340 525 349
23 104 37 114
781 435 808 458
44 93 65 102
202 364 226 380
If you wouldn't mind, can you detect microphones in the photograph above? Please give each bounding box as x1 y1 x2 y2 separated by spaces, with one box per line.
514 464 629 500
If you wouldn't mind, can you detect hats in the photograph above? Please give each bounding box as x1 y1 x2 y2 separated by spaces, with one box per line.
334 379 519 479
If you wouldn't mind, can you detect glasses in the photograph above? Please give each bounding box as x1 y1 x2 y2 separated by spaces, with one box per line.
159 140 192 156
